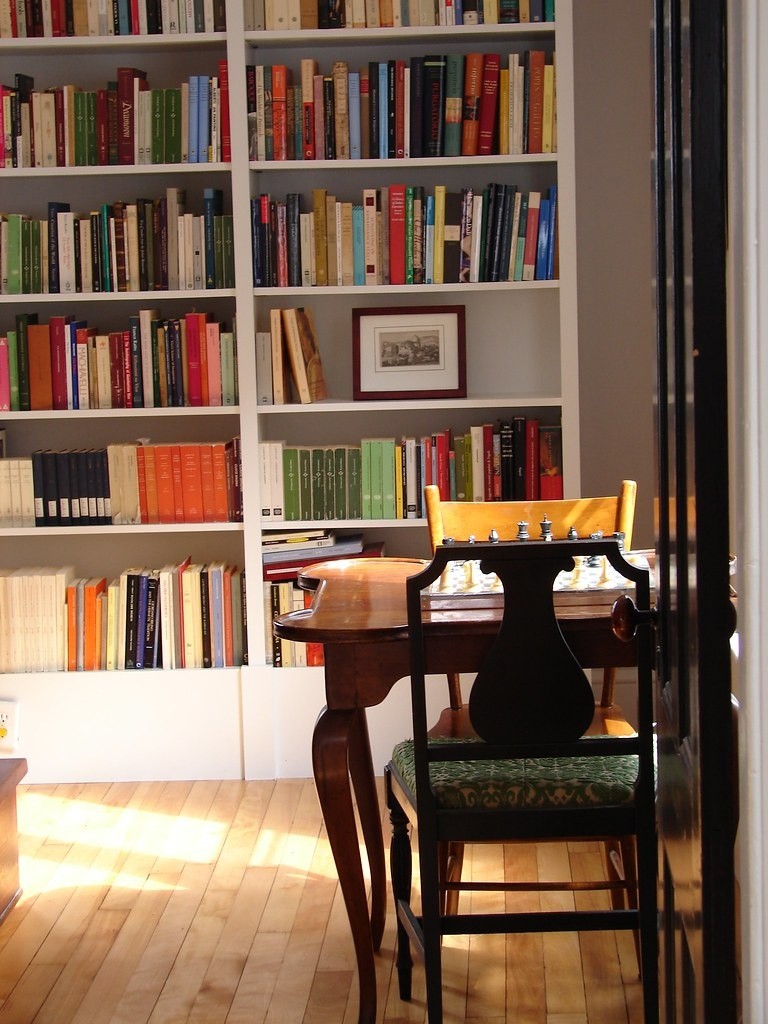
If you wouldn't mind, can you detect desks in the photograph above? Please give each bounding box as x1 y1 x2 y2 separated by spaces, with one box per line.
272 557 739 1024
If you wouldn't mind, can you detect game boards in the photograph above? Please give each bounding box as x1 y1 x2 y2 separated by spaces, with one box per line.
428 552 656 598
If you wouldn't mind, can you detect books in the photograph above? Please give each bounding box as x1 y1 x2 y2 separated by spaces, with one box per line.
0 0 562 673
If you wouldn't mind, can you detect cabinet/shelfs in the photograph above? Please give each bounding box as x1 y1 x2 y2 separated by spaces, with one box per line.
0 0 584 782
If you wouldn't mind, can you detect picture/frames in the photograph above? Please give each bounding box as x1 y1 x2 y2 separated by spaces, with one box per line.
353 304 468 399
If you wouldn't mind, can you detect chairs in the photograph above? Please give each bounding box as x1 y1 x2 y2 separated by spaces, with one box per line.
382 481 665 1020
